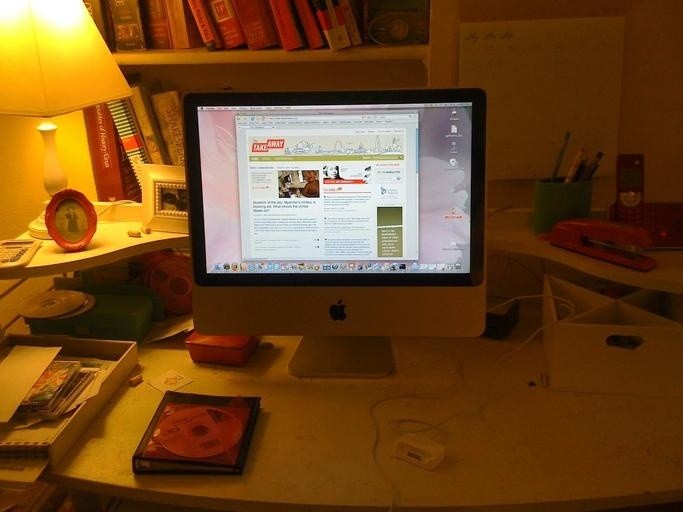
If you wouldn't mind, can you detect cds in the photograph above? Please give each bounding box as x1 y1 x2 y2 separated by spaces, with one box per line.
154 406 245 461
17 288 96 319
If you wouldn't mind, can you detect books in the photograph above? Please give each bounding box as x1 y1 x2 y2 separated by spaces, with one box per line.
152 90 182 163
16 361 96 421
132 390 262 476
128 80 169 164
83 106 141 201
109 99 150 192
88 0 363 51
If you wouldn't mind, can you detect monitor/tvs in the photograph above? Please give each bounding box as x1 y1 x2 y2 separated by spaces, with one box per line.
182 88 486 379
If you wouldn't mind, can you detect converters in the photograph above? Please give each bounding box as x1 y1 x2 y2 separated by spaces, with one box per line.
391 430 446 472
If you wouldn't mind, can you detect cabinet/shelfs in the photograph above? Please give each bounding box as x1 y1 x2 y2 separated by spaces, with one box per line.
0 204 683 512
84 1 432 201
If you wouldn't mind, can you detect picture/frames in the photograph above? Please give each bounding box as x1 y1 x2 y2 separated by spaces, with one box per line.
141 164 189 234
45 188 97 252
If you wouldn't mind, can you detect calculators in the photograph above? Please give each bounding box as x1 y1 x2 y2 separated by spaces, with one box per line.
0 239 41 269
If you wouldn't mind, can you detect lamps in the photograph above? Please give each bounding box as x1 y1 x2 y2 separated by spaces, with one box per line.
1 1 134 240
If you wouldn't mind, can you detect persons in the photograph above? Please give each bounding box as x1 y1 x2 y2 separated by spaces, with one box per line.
303 171 319 197
64 205 81 231
323 166 342 179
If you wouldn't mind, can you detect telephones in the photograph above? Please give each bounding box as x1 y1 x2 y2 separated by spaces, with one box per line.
607 154 683 250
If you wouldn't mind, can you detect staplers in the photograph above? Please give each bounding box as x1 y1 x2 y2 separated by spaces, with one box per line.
550 219 657 272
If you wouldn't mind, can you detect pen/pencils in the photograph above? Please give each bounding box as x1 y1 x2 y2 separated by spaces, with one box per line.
550 131 571 183
565 149 603 183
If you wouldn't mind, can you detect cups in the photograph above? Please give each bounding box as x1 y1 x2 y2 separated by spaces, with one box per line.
533 175 594 236
